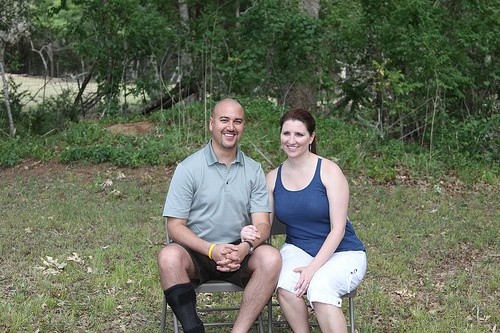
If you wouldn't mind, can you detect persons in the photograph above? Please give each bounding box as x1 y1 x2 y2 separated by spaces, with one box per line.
156 98 283 333
240 109 367 333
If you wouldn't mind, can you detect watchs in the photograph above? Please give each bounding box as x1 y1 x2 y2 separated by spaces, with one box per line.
242 240 255 252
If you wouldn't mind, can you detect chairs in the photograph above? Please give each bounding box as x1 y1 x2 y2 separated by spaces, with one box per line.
162 218 265 333
269 216 357 333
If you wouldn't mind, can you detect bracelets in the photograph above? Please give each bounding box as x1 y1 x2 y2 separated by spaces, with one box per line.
208 244 215 259
